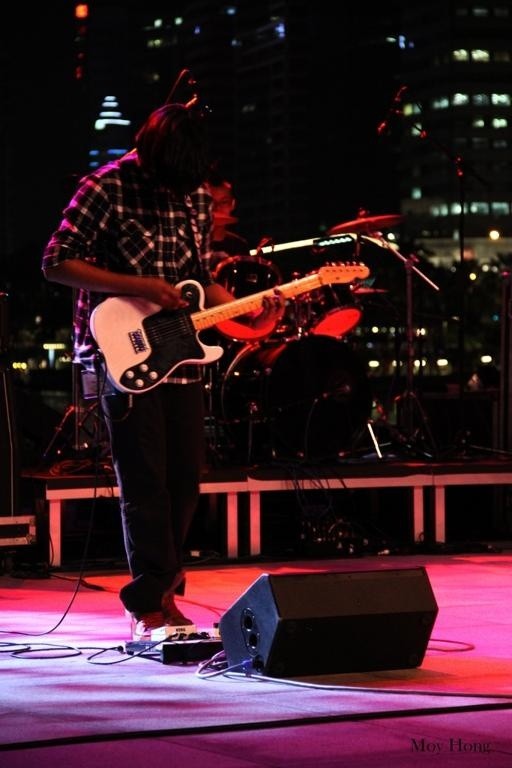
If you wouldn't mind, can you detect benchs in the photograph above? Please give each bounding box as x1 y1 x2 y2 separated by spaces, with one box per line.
38 459 512 565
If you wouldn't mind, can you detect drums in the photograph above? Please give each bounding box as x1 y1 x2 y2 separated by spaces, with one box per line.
292 268 364 340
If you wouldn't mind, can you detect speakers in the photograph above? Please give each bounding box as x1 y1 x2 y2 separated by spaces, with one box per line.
219 564 440 678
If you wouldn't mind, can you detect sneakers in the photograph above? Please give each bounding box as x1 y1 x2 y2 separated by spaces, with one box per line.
131 589 193 640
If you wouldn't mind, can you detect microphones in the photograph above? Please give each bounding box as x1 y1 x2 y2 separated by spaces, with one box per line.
378 90 405 133
189 80 206 121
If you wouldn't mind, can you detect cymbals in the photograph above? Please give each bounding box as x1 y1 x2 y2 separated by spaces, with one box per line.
347 282 388 297
333 214 403 238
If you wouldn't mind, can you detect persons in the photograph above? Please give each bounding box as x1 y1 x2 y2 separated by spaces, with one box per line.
468 366 500 390
41 103 285 641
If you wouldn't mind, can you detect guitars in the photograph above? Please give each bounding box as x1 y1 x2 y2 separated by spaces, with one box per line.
209 253 285 343
220 330 368 467
90 260 370 395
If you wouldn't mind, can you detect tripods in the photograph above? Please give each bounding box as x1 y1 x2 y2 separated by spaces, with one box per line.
36 264 118 474
357 233 443 461
